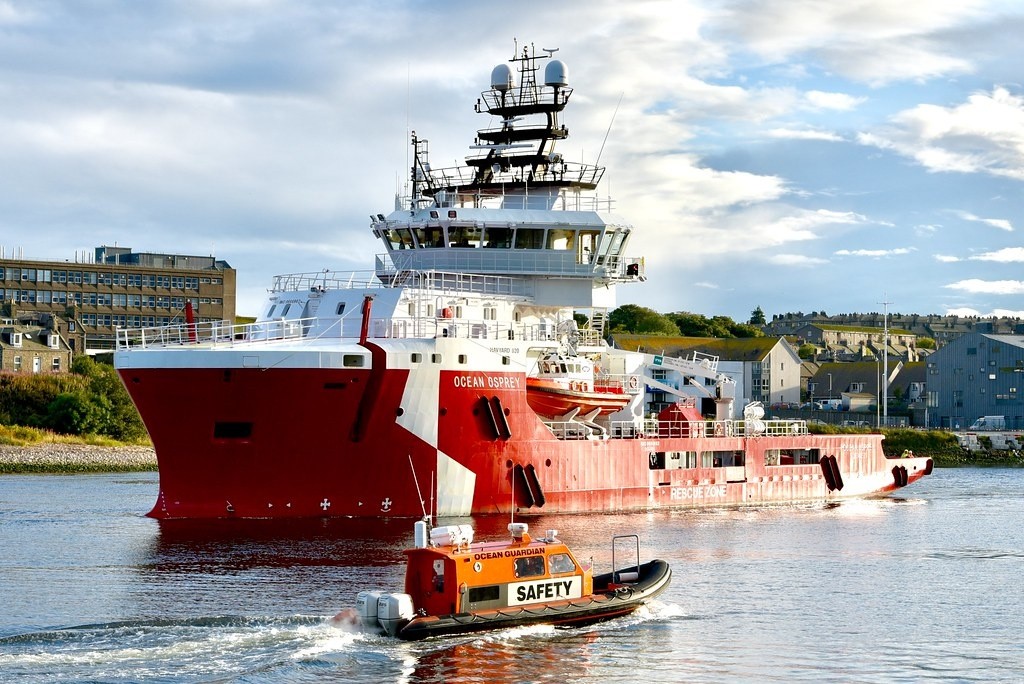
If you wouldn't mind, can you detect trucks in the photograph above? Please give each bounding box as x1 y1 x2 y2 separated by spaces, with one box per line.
970 415 1006 431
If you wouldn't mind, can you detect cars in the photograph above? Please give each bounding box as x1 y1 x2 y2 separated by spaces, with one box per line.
769 402 792 409
801 402 822 409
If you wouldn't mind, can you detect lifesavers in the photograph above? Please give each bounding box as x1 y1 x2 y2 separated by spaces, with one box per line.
630 376 638 388
570 381 580 391
580 381 589 392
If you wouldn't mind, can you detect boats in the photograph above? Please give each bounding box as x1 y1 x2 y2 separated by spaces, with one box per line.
326 455 671 647
113 37 934 519
